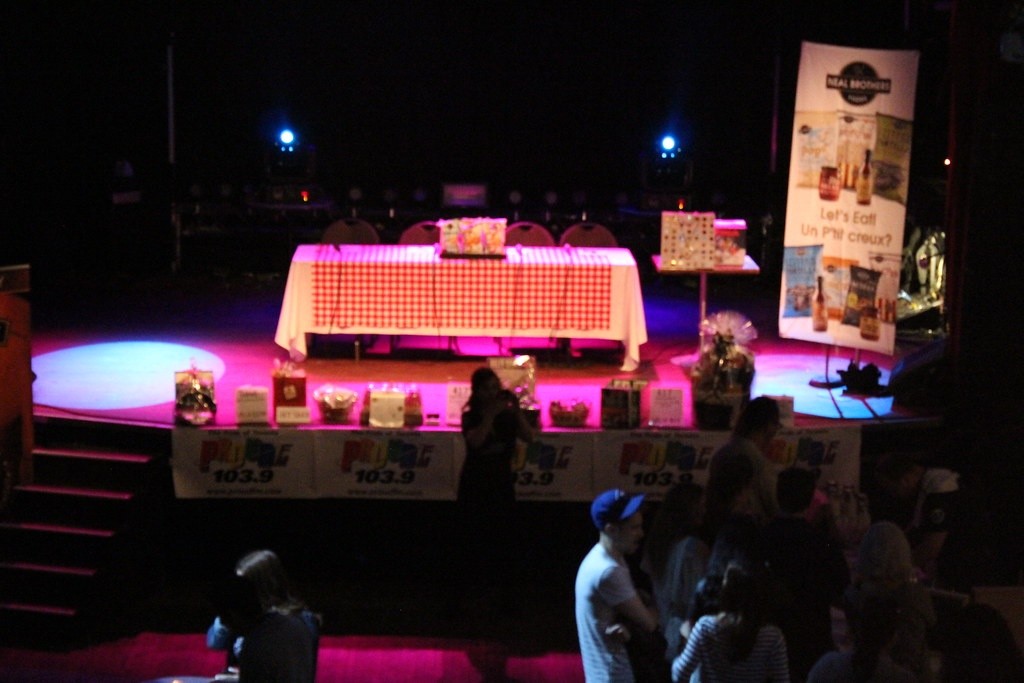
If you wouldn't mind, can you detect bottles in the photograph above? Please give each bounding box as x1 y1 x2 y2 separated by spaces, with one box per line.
819 167 841 200
856 149 873 205
812 276 828 332
861 307 881 341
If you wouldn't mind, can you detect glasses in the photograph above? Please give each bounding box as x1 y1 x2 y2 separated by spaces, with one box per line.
773 417 783 429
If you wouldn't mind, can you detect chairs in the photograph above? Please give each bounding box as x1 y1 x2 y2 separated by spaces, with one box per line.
507 221 560 248
323 218 381 243
558 223 619 247
401 221 441 244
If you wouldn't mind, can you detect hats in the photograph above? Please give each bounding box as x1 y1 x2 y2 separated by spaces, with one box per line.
590 488 646 529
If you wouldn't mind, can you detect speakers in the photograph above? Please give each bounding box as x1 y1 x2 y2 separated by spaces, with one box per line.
889 337 948 409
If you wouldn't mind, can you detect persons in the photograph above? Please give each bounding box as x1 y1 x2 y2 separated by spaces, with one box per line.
457 368 536 562
572 398 1024 683
207 548 318 683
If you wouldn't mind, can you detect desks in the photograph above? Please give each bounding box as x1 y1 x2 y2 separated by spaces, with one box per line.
653 253 759 367
276 243 647 371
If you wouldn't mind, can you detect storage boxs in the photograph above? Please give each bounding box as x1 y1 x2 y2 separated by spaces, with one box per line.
600 389 640 428
660 210 750 271
